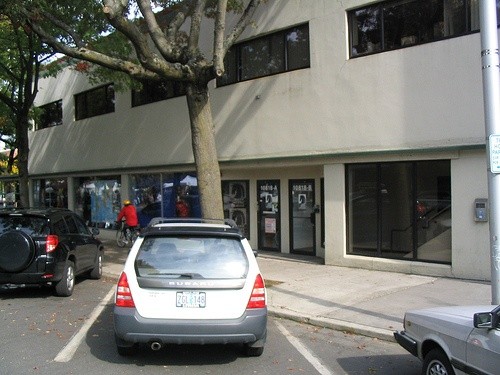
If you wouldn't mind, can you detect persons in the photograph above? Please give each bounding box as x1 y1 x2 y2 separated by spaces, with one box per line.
118 200 138 244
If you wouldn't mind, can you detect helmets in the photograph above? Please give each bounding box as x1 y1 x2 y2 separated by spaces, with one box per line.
123 200 131 205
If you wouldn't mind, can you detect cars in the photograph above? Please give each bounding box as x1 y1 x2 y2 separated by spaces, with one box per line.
113 216 267 358
394 303 500 375
350 193 427 254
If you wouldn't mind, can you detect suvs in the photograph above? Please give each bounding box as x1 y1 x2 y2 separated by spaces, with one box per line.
0 208 106 298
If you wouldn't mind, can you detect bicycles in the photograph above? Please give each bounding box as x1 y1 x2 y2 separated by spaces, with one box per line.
114 219 144 246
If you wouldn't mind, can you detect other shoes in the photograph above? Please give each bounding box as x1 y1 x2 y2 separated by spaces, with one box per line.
122 240 129 245
